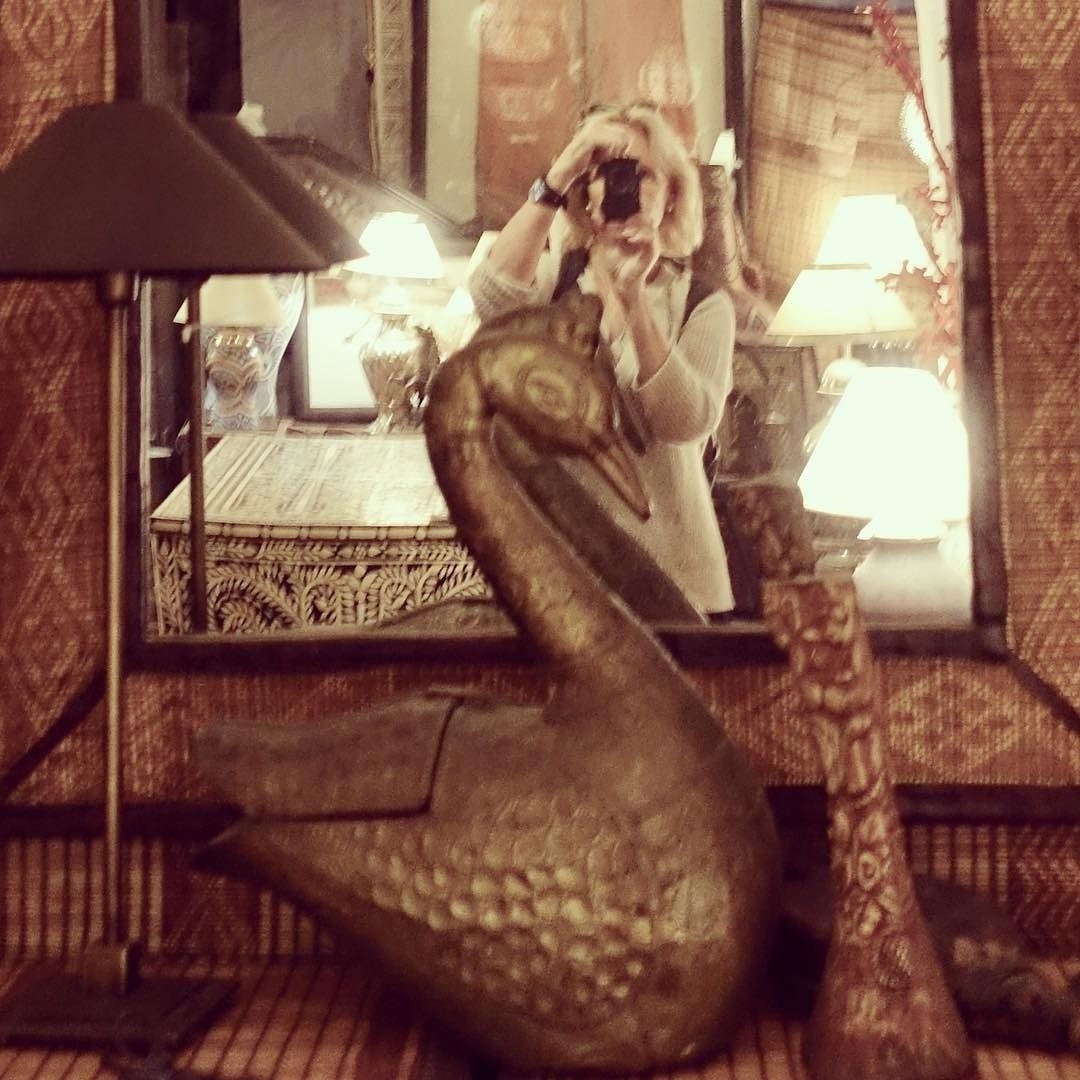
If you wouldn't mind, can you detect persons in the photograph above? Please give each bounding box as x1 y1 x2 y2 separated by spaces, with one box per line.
468 103 742 622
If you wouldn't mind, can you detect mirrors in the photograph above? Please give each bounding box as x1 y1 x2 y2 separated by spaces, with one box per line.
110 0 1009 668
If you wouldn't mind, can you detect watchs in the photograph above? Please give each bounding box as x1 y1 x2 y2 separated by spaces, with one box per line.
526 176 565 213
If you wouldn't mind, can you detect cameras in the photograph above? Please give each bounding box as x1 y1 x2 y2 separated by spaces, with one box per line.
604 156 640 223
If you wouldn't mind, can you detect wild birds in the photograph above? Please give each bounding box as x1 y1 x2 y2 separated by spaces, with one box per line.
171 334 783 1071
349 288 711 636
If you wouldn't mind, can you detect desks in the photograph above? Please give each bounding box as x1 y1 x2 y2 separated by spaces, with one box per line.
147 433 487 633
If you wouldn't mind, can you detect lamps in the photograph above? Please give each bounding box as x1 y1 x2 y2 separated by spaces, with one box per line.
176 113 368 632
2 98 336 1066
344 217 460 435
763 191 972 616
